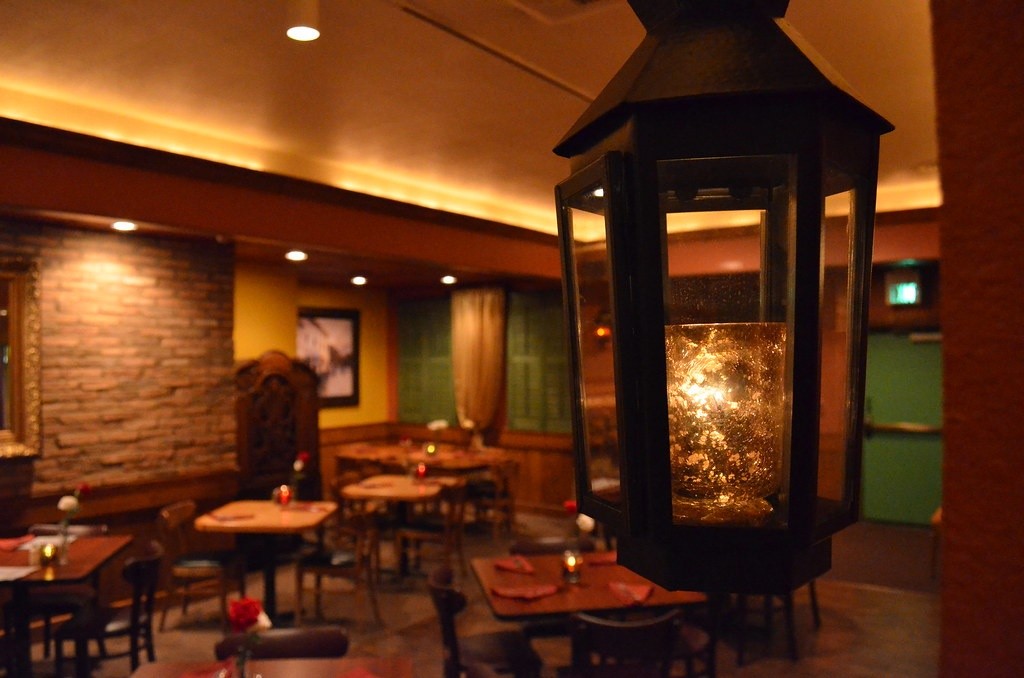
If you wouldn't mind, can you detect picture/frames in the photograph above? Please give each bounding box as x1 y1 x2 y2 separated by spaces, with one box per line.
296 307 360 407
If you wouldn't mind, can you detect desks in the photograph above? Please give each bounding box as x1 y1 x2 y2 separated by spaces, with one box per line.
131 657 416 678
0 534 132 678
337 444 491 541
470 551 708 665
195 500 338 628
341 475 441 585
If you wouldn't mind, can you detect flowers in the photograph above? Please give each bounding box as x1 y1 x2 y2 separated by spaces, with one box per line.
229 598 264 678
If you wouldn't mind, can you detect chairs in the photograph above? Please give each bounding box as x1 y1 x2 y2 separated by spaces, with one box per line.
0 460 821 678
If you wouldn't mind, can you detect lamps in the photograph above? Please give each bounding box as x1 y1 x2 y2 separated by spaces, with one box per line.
552 0 895 593
